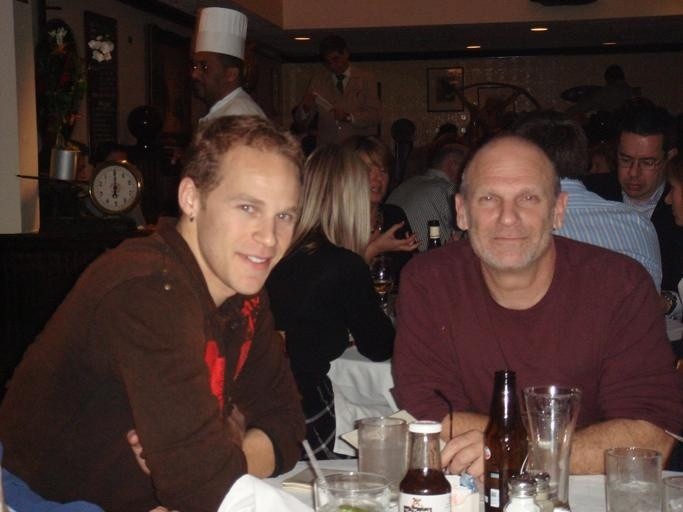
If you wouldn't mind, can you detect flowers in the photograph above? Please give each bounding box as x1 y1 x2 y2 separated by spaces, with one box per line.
47 28 114 145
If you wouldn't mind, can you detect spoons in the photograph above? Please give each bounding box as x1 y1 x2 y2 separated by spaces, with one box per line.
302 440 338 510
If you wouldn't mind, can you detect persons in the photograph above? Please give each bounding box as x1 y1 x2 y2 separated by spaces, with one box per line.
517 109 661 296
292 35 381 144
172 7 276 166
262 144 397 460
601 114 683 291
341 135 422 299
661 151 683 325
382 141 468 247
585 141 619 178
391 134 680 480
0 115 308 512
291 101 324 155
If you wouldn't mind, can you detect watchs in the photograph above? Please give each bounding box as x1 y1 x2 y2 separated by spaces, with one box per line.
664 287 678 316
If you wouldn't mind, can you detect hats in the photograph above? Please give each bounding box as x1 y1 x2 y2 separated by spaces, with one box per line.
189 15 248 57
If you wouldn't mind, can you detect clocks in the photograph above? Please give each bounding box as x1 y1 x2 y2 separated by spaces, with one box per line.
88 159 145 218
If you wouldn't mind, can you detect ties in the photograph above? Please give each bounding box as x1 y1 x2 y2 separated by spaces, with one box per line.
335 74 346 95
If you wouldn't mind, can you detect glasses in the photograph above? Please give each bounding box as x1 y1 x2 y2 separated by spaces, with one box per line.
616 155 655 169
432 388 453 471
188 62 229 72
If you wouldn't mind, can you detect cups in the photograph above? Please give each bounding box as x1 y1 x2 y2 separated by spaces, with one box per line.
524 385 580 511
357 418 405 507
604 448 663 511
663 476 683 512
313 473 392 511
371 257 395 308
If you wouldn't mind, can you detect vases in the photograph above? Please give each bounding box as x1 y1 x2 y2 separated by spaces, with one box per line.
49 148 81 181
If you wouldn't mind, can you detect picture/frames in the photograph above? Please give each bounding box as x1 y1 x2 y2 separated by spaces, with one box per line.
426 65 465 113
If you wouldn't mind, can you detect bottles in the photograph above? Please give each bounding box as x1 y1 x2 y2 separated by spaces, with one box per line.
427 220 443 250
533 472 554 512
505 472 543 512
399 419 451 511
482 369 532 512
